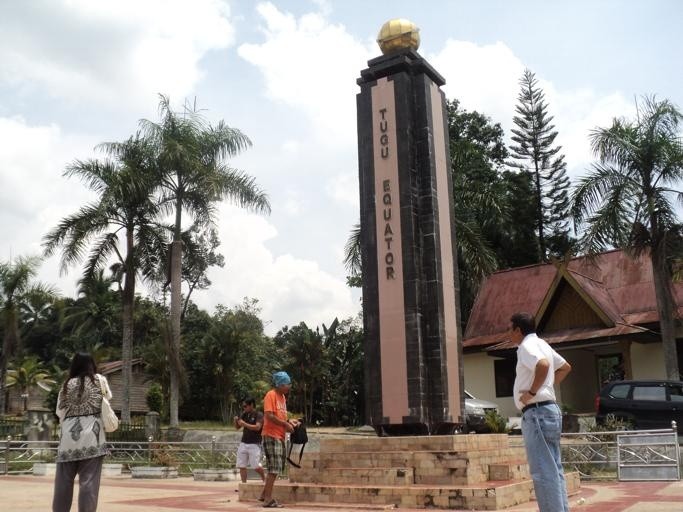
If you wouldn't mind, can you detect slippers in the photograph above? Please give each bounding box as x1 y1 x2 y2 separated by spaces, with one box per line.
258 496 284 508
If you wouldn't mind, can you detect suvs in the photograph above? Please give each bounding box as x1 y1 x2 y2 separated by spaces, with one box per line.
593 379 682 436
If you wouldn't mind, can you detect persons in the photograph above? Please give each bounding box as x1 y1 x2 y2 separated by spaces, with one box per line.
233 395 266 483
50 350 113 512
256 371 301 508
603 364 626 384
507 312 572 512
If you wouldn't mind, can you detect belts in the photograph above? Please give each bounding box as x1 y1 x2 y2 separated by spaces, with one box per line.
522 401 555 412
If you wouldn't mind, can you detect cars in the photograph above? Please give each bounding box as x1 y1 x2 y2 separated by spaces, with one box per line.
463 389 498 432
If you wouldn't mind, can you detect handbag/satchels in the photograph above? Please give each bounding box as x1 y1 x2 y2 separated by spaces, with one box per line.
102 396 120 433
290 422 308 443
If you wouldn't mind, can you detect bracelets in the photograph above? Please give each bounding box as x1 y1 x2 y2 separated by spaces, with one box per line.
529 389 537 396
